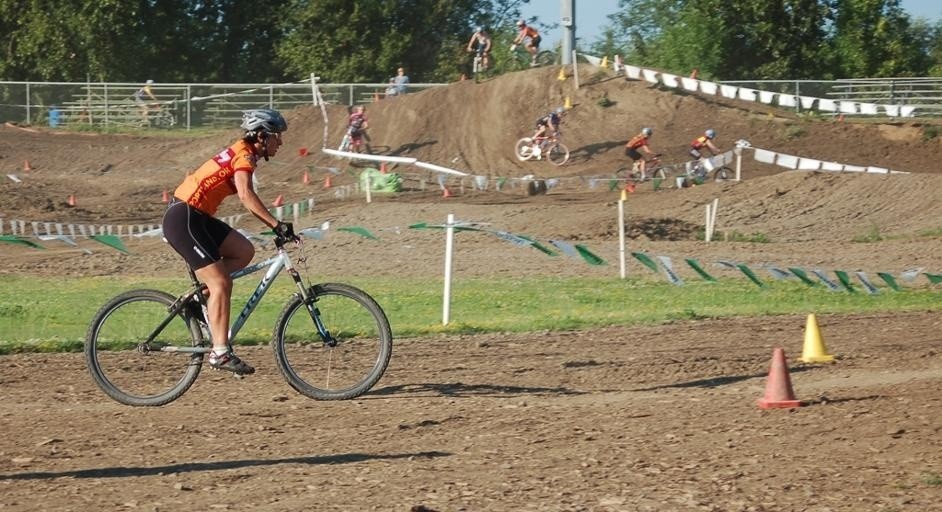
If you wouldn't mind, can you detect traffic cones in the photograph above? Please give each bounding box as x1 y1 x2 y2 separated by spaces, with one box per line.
839 112 846 122
67 195 78 204
600 56 609 68
379 164 387 174
562 96 574 108
795 314 834 363
689 70 697 80
162 191 170 201
619 189 629 202
557 69 567 81
272 195 283 208
323 176 333 187
768 110 776 117
302 171 311 183
23 159 31 171
756 348 801 407
613 56 624 69
442 187 455 197
375 90 381 102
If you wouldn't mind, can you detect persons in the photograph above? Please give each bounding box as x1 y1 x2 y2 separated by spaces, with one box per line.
688 128 721 159
624 127 658 181
163 109 294 374
527 107 568 149
467 27 492 72
345 104 369 167
513 19 541 64
385 68 409 95
132 80 160 125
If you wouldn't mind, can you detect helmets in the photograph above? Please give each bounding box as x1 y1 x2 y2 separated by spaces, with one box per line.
556 106 566 113
641 128 652 138
240 108 288 133
515 19 526 26
704 129 716 139
357 104 366 110
476 26 483 32
397 67 405 72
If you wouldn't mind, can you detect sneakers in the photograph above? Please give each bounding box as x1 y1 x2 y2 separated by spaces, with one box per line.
208 349 255 375
180 294 208 326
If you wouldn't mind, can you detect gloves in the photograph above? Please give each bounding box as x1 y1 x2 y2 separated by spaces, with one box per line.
273 220 295 238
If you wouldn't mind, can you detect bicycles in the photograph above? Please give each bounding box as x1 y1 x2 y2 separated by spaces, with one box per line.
514 128 569 167
501 47 555 75
677 150 735 188
124 98 174 130
613 153 677 191
347 126 367 166
468 48 496 83
84 222 394 407
